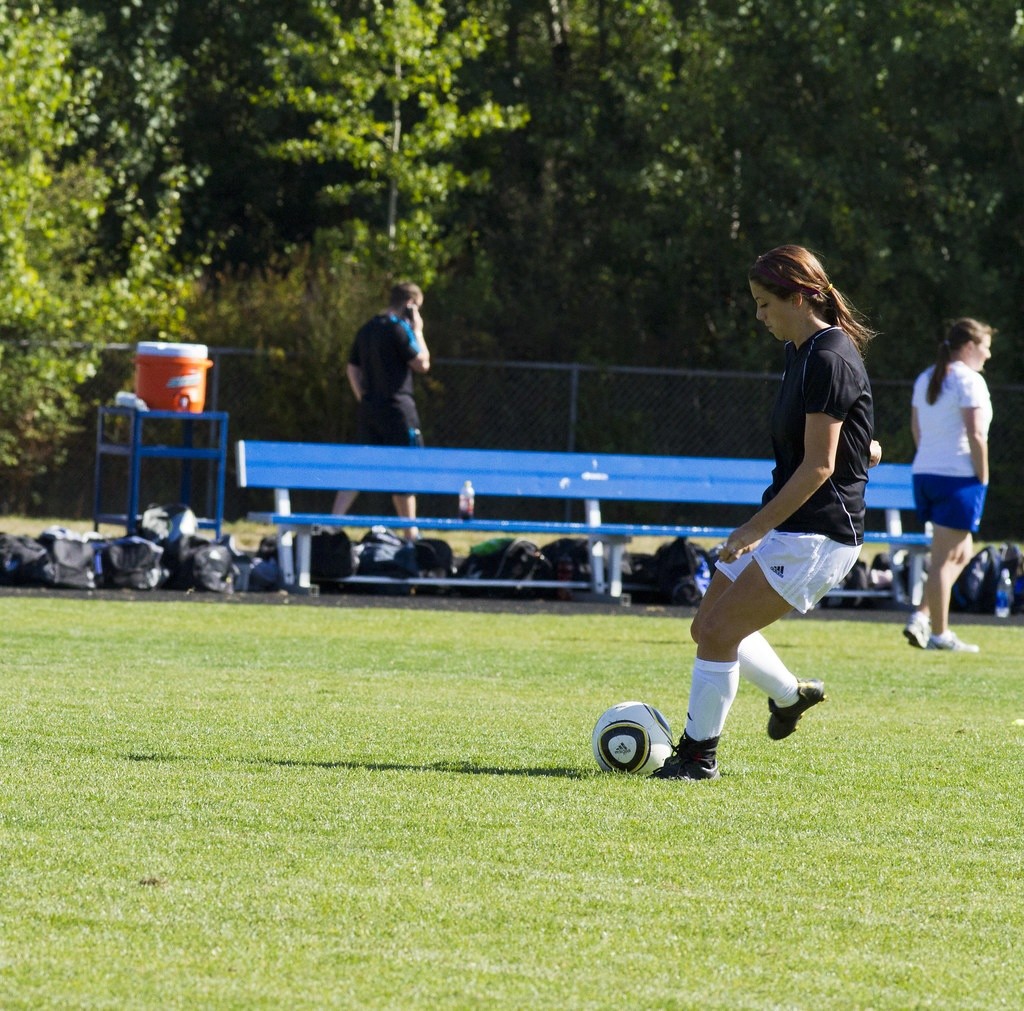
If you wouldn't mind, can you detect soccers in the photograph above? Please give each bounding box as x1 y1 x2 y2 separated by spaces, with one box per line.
591 702 673 775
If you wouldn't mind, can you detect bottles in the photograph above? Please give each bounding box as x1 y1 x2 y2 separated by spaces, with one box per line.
994 568 1014 617
459 480 476 520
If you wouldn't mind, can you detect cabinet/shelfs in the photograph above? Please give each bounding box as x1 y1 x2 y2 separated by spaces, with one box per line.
93 406 230 542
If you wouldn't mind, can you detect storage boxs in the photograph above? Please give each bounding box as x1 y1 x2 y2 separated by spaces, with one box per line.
130 340 215 414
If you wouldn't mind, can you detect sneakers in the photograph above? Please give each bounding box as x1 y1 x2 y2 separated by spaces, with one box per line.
646 729 721 781
901 610 980 654
766 675 826 739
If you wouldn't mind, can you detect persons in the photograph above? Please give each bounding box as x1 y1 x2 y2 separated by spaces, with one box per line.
641 240 884 783
899 314 996 654
332 279 434 544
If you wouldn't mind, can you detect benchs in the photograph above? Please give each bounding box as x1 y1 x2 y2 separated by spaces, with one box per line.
238 437 937 607
